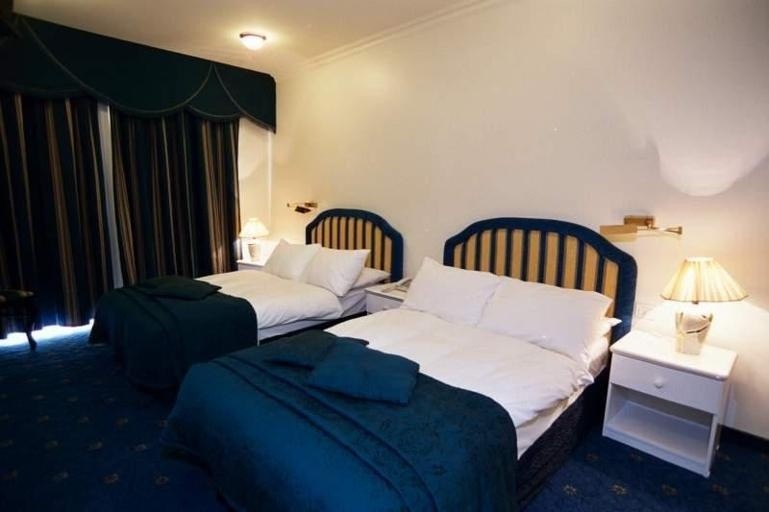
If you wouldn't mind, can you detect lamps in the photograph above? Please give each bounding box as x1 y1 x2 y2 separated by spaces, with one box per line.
240 34 266 49
287 203 317 214
660 256 749 355
238 218 270 262
599 217 682 236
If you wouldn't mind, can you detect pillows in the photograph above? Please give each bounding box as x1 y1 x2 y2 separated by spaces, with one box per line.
309 339 419 405
151 277 221 301
263 238 391 298
269 330 369 368
138 277 166 288
401 256 622 369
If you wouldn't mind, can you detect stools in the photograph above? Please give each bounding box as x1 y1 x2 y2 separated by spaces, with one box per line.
0 289 38 349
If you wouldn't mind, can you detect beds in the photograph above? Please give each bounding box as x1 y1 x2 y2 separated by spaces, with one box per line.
88 208 403 390
160 218 637 512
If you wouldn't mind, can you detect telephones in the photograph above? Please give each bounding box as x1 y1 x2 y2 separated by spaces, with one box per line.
395 277 412 292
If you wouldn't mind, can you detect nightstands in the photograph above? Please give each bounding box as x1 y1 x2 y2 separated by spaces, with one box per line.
365 281 406 314
602 328 738 479
237 261 263 270
